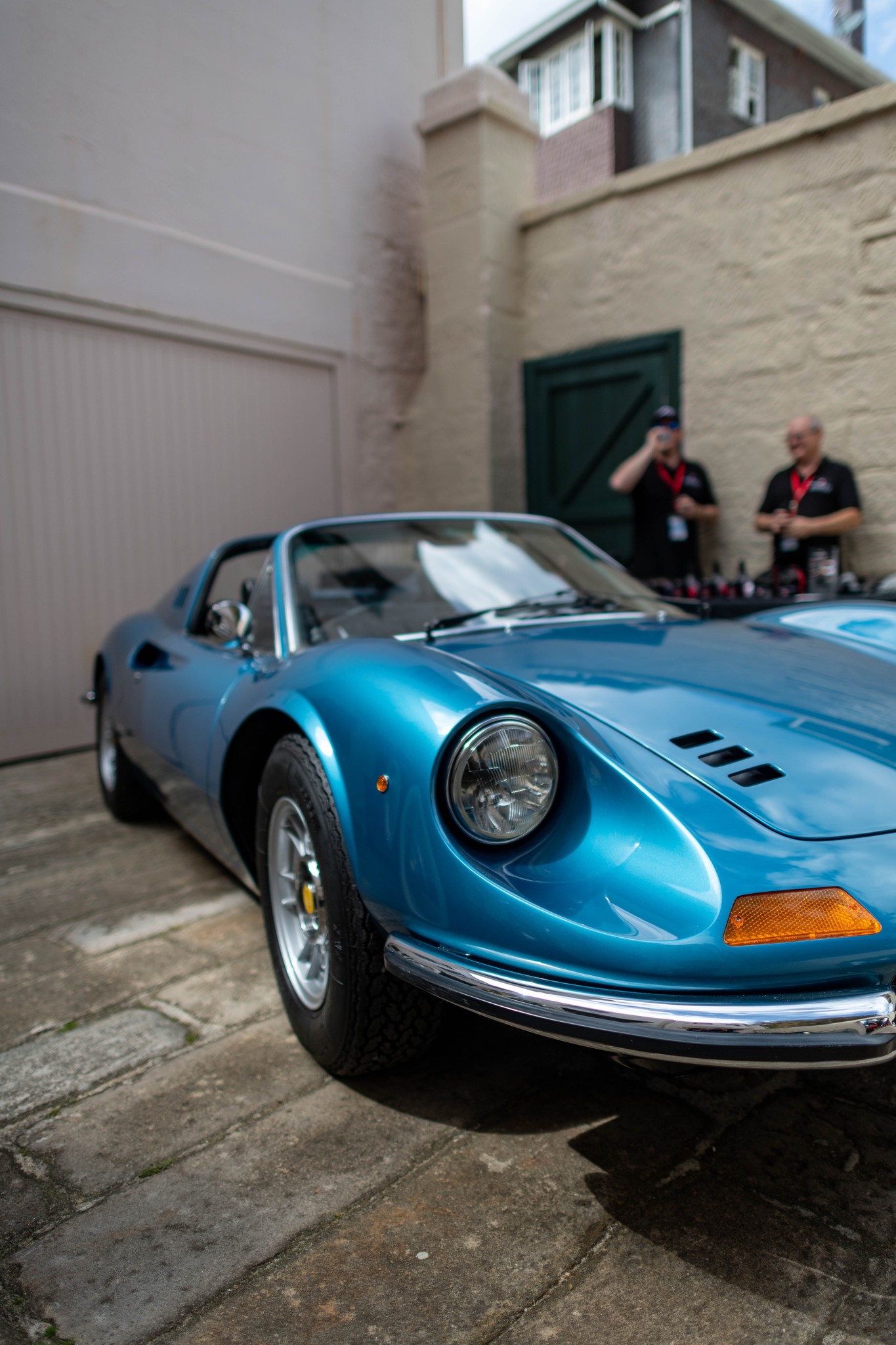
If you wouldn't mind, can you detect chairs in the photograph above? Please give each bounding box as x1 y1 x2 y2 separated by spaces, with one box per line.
242 578 327 647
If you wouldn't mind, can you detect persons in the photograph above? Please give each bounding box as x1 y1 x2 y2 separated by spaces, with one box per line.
753 415 861 594
610 406 719 596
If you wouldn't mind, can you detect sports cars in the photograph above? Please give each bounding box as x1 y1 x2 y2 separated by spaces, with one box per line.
80 507 896 1085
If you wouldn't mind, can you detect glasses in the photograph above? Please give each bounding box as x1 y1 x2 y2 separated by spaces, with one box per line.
787 429 815 443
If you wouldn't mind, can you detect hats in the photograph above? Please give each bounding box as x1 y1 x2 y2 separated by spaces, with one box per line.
649 405 679 430
